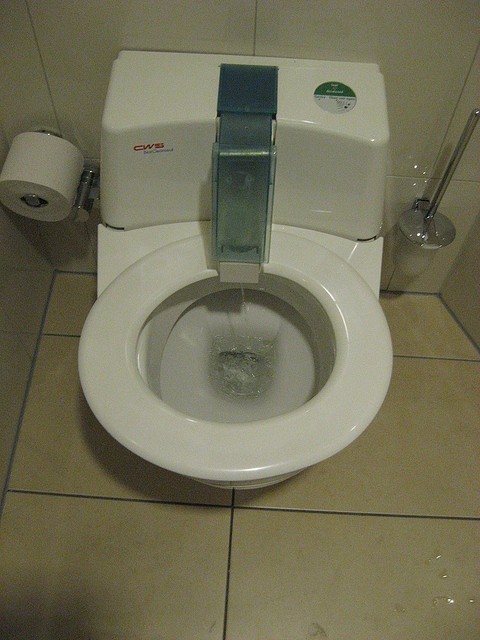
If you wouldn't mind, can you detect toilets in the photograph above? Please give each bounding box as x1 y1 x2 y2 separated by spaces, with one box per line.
77 48 394 489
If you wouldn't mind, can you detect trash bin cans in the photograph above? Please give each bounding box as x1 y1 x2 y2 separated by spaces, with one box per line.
392 109 480 274
